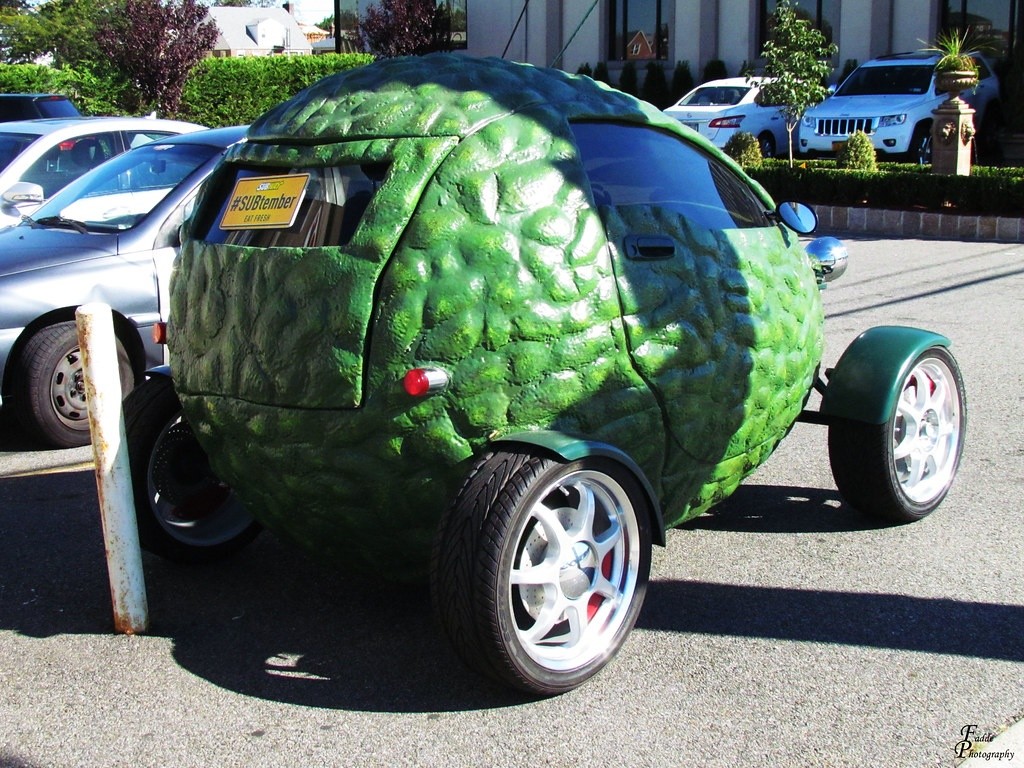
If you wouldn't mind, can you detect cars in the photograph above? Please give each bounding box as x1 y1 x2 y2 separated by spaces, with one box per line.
661 75 826 156
0 94 254 452
165 51 967 701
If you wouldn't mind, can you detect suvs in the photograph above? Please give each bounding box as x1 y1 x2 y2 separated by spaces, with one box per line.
796 47 1003 164
